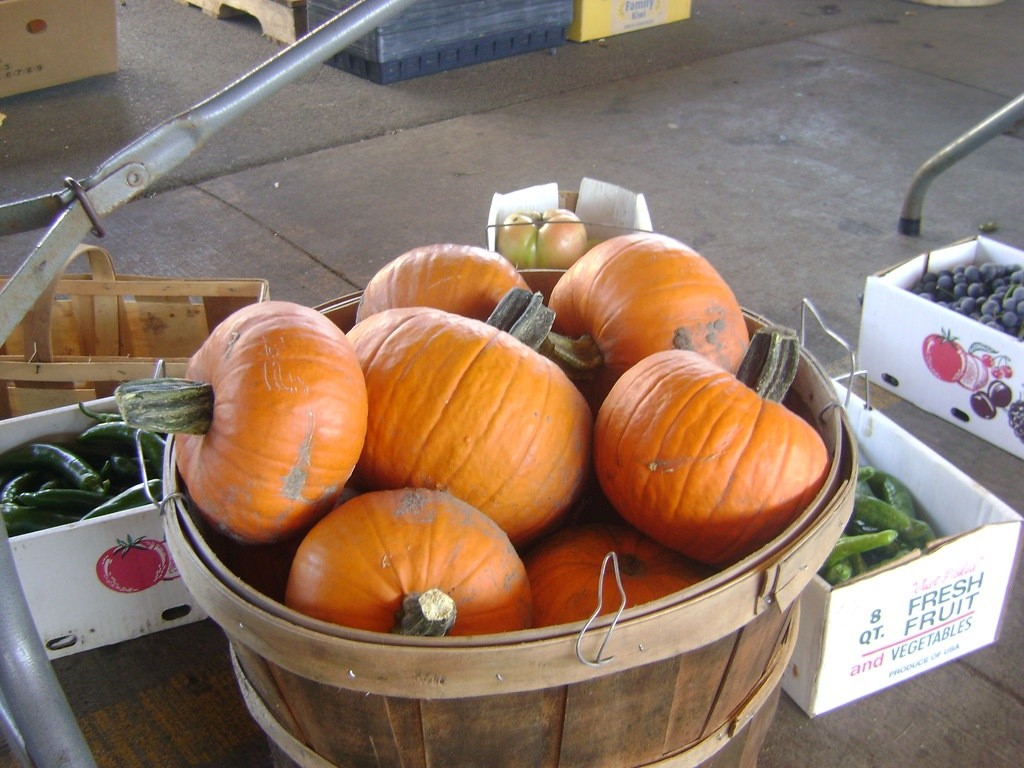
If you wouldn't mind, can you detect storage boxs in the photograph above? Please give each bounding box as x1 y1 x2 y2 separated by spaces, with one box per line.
860 235 1024 456
0 398 210 660
782 379 1022 719
137 264 861 768
486 178 654 261
307 0 572 84
566 1 690 42
1 0 118 97
0 243 267 418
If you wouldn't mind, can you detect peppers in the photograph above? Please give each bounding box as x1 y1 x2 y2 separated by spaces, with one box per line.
1 401 165 534
819 467 939 584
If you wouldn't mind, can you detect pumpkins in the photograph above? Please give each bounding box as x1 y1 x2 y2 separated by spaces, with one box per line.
112 232 829 637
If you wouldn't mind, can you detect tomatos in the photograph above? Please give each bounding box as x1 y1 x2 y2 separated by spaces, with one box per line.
496 209 588 270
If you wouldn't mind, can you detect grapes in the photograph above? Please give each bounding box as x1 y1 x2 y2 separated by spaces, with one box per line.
911 263 1024 345
